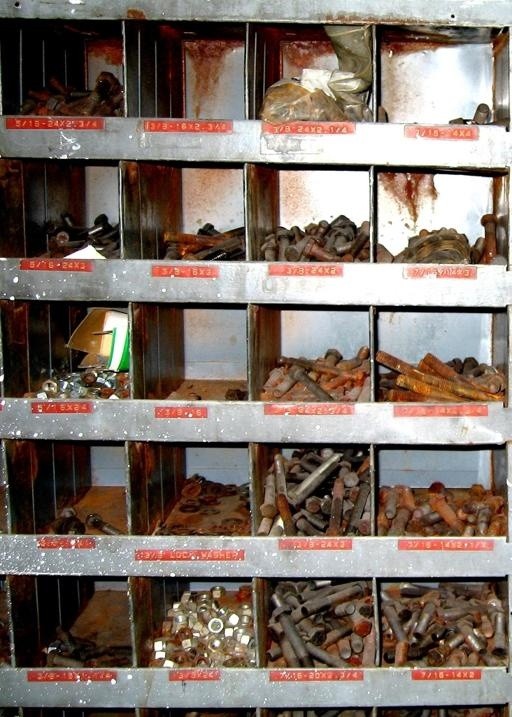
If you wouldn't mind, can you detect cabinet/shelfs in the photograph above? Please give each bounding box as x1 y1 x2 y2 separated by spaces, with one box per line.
0 1 509 717
